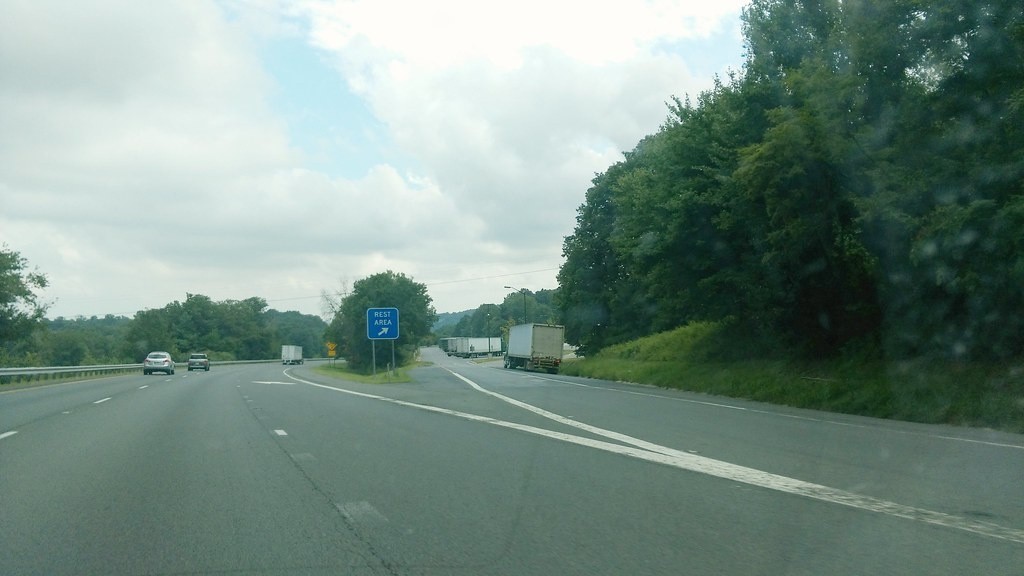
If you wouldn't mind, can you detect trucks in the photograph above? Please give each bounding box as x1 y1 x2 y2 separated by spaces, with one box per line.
282 345 304 365
503 323 565 374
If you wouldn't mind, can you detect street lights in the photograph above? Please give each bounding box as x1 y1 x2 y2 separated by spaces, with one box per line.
504 286 526 323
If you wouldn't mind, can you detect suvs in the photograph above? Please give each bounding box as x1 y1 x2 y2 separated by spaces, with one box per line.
144 351 175 375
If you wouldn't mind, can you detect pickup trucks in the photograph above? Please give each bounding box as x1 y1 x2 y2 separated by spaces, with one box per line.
188 354 210 371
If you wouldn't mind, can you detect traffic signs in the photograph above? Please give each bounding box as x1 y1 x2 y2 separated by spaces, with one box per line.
367 308 400 339
